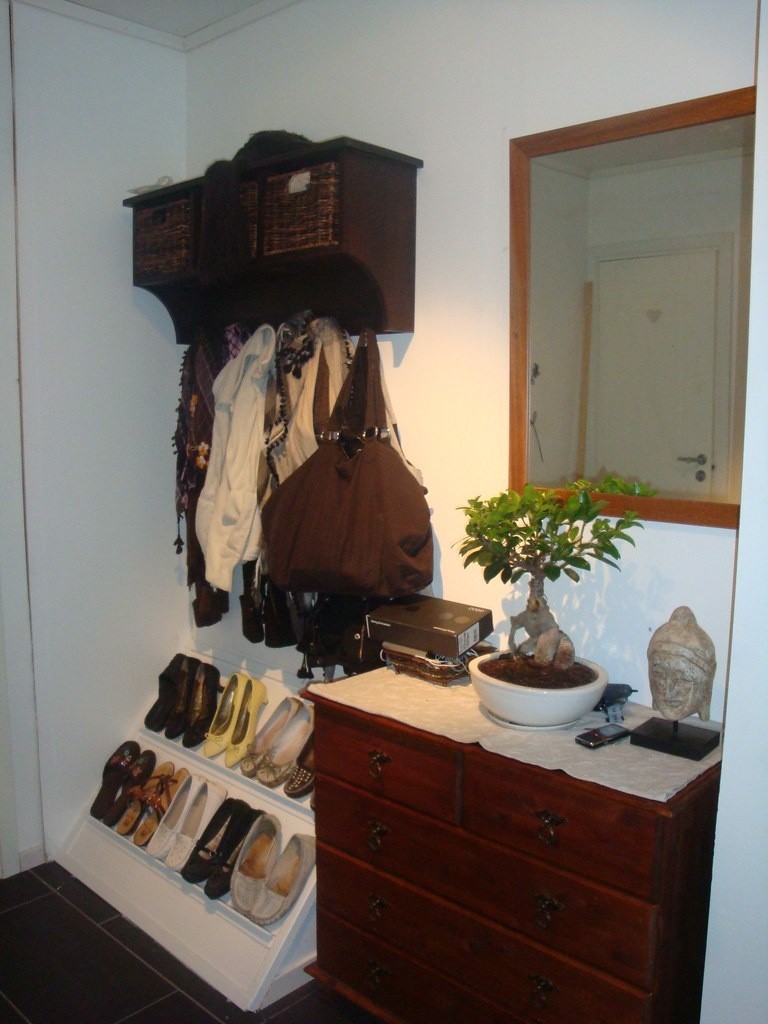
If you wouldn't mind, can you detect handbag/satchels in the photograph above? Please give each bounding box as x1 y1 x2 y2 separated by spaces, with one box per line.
261 328 435 598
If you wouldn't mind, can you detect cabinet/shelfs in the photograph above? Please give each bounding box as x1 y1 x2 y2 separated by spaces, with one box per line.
53 651 319 1012
123 136 424 345
301 659 722 1024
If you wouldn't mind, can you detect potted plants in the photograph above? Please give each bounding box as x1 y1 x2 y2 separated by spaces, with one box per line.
456 484 643 732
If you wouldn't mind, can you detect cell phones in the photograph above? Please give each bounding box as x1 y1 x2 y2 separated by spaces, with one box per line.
575 723 630 749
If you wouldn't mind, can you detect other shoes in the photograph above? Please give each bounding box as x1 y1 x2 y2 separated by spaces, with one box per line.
181 797 268 899
145 654 187 732
284 728 315 812
240 698 315 789
145 774 228 872
229 813 316 925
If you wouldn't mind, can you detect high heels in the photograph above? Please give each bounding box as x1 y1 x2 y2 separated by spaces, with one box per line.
204 673 268 769
165 657 226 748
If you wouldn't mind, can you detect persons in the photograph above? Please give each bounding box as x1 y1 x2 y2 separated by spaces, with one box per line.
647 606 717 722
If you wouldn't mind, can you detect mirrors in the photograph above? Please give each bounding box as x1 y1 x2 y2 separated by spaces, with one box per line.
508 86 757 529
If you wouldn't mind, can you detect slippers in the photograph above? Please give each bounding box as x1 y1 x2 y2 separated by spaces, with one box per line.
90 741 156 826
118 762 189 847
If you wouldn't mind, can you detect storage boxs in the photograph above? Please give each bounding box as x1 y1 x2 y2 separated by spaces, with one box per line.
364 594 494 658
